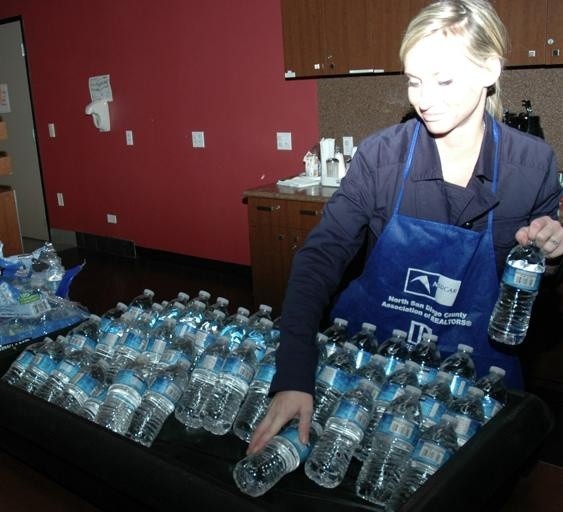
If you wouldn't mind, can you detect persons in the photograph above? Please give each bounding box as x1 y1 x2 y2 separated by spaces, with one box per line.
243 0 563 455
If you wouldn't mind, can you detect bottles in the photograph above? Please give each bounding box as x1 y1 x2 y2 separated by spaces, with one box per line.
2 287 508 512
0 233 78 348
487 238 545 346
326 146 351 178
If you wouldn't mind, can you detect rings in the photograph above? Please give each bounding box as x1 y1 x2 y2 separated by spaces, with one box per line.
549 238 559 247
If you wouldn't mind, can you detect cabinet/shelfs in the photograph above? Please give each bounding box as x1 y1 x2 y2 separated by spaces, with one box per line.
280 0 562 81
246 196 325 318
